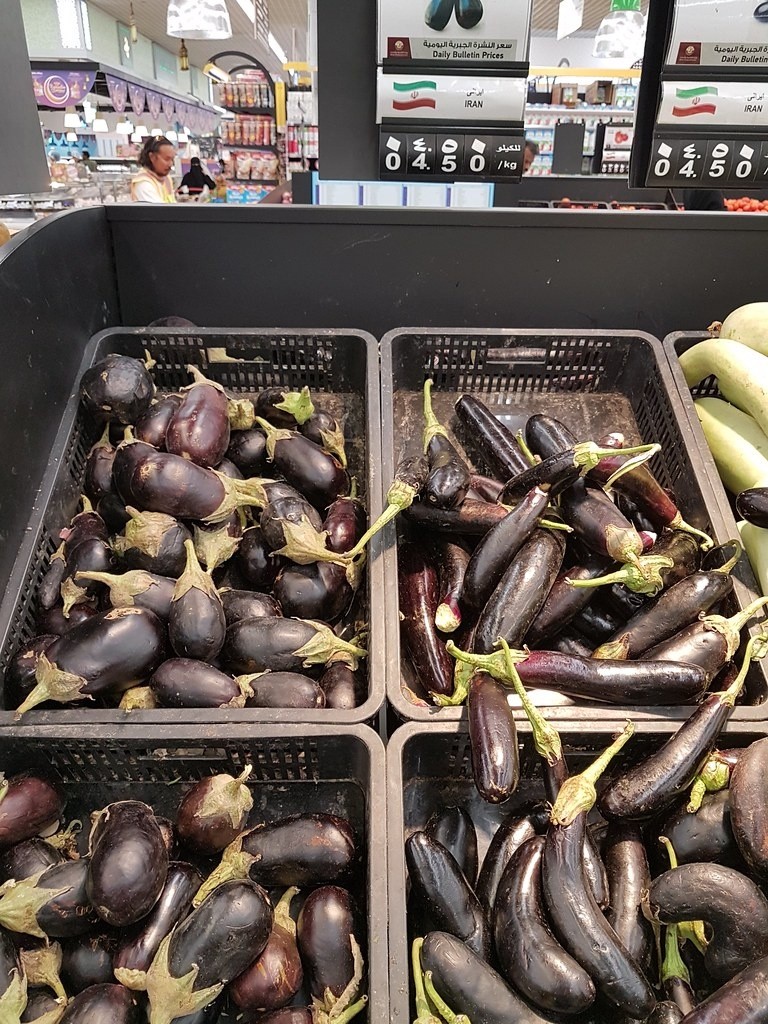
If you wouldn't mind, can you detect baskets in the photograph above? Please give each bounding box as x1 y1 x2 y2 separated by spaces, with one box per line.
0 327 768 1024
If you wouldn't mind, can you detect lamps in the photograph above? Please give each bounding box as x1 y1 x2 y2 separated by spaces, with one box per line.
67 127 78 141
592 0 647 57
166 0 234 40
93 82 109 132
166 124 178 141
136 116 148 137
178 125 190 144
64 105 82 127
117 112 129 136
153 121 163 137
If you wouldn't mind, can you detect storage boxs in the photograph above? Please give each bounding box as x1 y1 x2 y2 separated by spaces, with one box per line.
524 107 635 157
381 327 768 721
0 324 377 726
387 720 768 1024
663 330 768 633
0 722 391 1024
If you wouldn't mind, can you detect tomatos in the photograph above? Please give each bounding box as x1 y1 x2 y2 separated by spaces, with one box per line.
724 197 768 213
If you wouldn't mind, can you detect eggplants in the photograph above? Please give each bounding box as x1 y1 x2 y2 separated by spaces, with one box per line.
0 348 767 1023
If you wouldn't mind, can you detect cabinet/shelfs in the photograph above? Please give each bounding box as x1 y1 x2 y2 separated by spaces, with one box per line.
209 50 281 186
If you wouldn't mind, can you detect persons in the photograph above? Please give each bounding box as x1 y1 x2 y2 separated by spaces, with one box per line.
130 135 176 204
523 139 540 175
178 156 229 200
68 148 98 172
